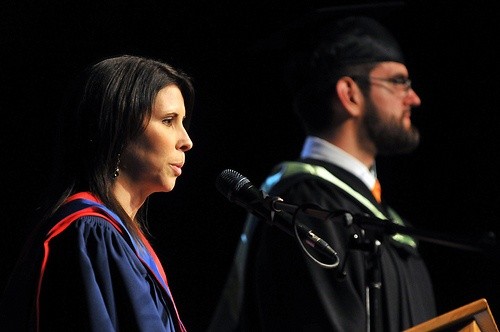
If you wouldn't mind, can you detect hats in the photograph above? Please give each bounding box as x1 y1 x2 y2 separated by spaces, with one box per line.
283 2 403 66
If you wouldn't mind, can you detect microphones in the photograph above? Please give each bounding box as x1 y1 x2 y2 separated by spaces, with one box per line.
217 169 338 261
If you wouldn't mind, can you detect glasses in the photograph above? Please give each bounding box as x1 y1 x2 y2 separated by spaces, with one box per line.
352 74 411 89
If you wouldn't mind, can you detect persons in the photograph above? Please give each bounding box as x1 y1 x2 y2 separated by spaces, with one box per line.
209 35 438 332
26 57 194 332
